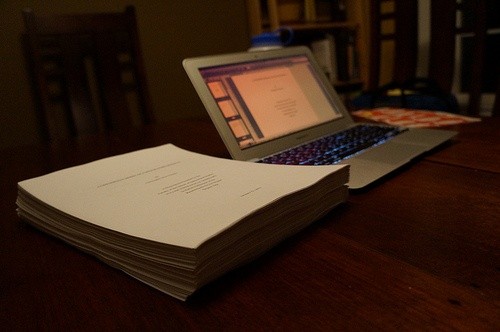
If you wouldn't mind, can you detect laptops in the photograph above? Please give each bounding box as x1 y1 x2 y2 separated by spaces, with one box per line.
182 45 459 191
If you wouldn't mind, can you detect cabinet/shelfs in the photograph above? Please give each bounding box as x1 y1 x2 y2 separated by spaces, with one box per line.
248 1 398 92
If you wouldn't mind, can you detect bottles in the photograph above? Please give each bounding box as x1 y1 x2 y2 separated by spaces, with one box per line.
248 33 283 51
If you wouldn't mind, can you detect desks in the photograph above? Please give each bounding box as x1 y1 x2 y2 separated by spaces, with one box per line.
0 102 500 332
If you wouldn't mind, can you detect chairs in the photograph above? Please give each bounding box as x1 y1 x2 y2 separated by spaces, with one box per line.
20 6 157 135
